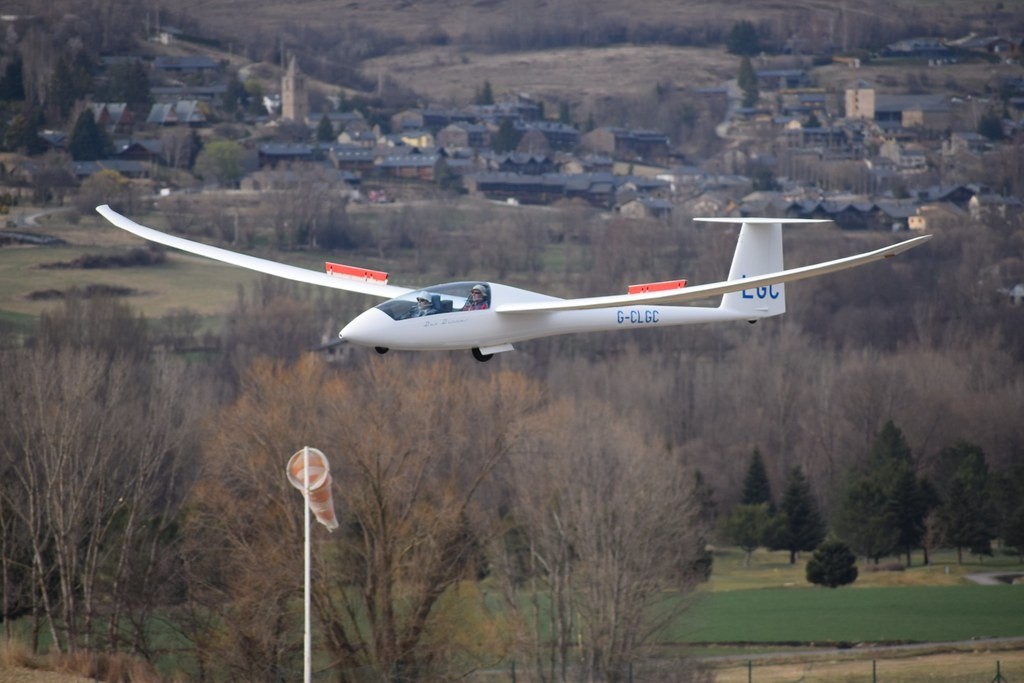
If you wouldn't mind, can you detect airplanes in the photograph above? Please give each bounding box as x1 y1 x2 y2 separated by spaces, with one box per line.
95 204 936 362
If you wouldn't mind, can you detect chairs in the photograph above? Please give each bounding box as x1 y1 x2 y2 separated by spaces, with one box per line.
438 299 452 313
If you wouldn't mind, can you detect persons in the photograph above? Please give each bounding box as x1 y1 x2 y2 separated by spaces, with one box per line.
463 285 488 311
413 291 437 317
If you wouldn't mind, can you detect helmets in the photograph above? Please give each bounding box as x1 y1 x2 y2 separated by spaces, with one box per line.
473 285 487 296
416 291 432 303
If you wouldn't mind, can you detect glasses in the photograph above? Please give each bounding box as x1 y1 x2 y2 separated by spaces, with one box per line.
417 298 428 302
471 291 480 294
